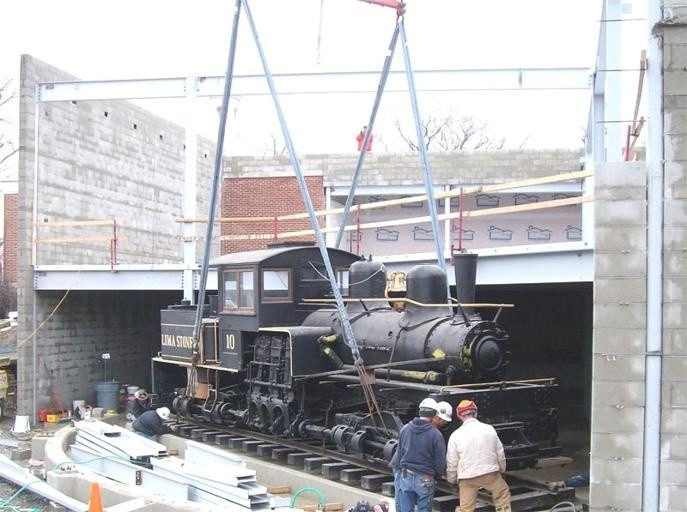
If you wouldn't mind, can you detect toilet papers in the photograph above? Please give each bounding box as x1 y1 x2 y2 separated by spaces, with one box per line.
14 415 30 433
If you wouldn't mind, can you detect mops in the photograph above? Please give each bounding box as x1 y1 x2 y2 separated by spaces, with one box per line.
40 362 73 424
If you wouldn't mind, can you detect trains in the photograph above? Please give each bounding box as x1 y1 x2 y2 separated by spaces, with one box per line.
150 239 572 483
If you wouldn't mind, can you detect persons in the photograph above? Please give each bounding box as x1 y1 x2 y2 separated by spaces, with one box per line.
355 126 373 151
444 397 513 511
547 471 588 491
387 400 452 512
131 406 172 442
396 397 447 511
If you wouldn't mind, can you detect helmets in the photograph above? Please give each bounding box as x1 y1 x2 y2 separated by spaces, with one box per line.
157 407 170 420
437 401 453 422
419 398 439 412
457 399 477 413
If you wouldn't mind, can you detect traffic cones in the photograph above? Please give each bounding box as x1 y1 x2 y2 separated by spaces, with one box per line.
83 482 106 512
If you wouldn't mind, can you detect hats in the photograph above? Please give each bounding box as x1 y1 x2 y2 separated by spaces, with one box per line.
135 388 149 401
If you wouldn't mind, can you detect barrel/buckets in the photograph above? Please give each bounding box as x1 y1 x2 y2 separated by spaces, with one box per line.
73 400 85 417
95 381 120 414
